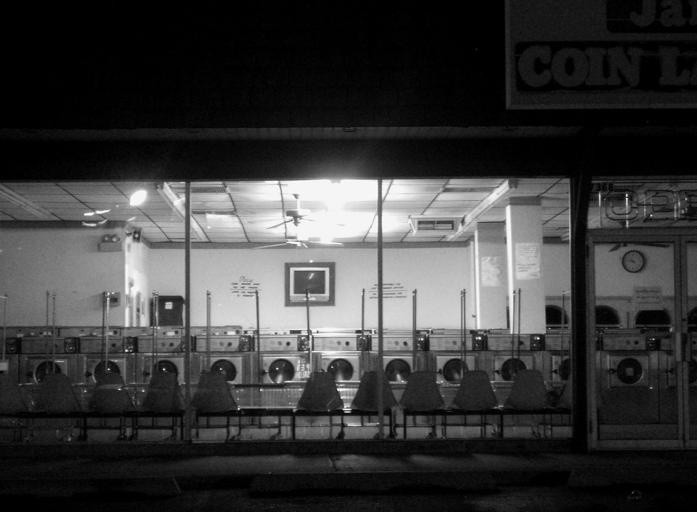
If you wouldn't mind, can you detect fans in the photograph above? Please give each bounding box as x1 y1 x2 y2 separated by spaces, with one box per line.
252 192 346 250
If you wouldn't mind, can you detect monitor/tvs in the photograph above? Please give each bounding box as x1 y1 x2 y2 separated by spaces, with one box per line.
289 267 330 302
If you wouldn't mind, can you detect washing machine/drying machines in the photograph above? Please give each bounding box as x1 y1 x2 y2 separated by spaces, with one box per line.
544 297 694 425
4 327 544 420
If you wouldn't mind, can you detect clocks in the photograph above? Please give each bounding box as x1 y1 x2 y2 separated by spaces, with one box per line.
622 250 645 273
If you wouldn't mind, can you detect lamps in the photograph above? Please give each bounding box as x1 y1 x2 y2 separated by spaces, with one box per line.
98 234 122 251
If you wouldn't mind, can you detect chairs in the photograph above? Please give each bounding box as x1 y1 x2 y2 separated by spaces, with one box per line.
0 365 574 442
